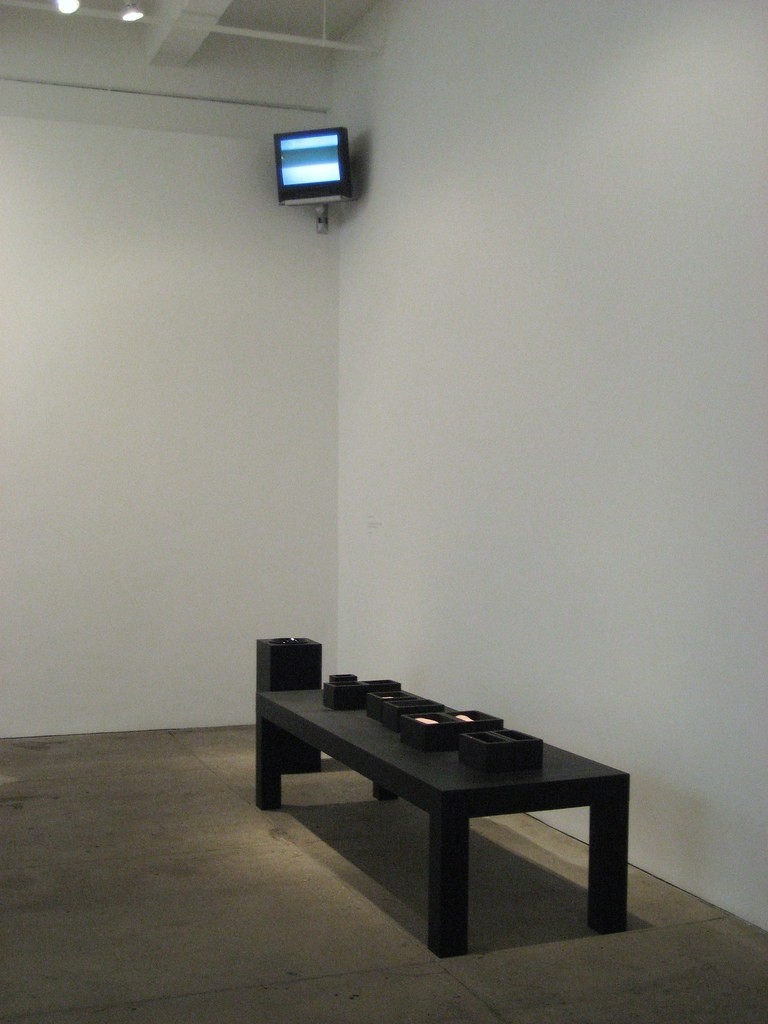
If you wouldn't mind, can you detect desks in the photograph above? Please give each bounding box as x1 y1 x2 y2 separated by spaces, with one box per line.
258 688 632 957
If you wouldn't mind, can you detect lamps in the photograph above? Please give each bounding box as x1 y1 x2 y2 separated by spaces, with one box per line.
122 4 144 23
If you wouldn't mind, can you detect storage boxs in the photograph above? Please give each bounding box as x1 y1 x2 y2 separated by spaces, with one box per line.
382 698 446 736
490 729 544 772
361 679 401 710
321 682 363 711
368 690 421 722
329 674 357 683
458 732 517 773
400 711 465 753
447 709 504 733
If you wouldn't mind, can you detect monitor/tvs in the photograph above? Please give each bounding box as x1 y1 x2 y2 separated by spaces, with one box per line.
273 126 351 206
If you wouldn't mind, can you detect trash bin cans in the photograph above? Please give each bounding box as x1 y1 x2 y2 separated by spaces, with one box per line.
257 636 323 773
